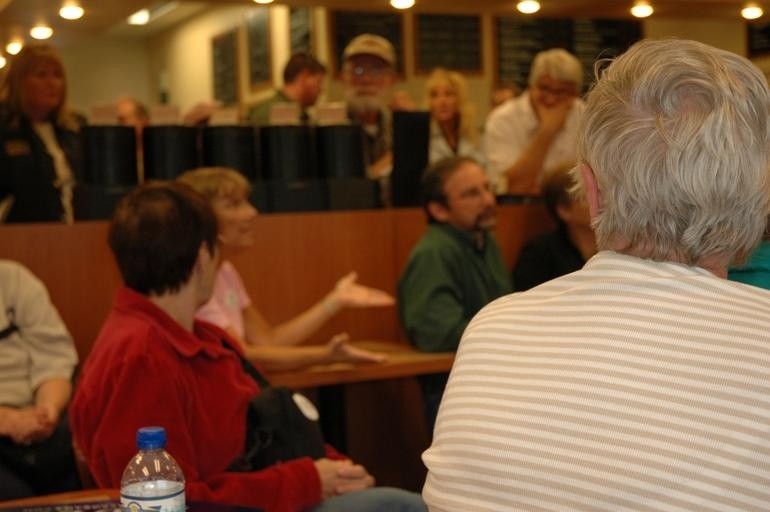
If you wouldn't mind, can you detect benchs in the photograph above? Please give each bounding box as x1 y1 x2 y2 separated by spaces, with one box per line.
0 203 562 491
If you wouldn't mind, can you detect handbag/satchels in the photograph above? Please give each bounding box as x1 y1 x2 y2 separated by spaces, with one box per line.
248 386 325 473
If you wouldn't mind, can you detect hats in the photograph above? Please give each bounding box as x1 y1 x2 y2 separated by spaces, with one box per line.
342 34 396 68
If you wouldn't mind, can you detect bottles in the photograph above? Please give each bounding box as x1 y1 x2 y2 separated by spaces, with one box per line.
120 427 186 512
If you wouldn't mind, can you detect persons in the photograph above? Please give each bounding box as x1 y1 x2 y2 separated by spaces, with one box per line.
419 37 770 511
394 154 509 436
423 48 589 202
0 42 110 225
177 168 396 372
0 258 95 502
510 162 597 290
114 96 153 184
245 50 327 125
68 178 427 511
343 33 417 208
